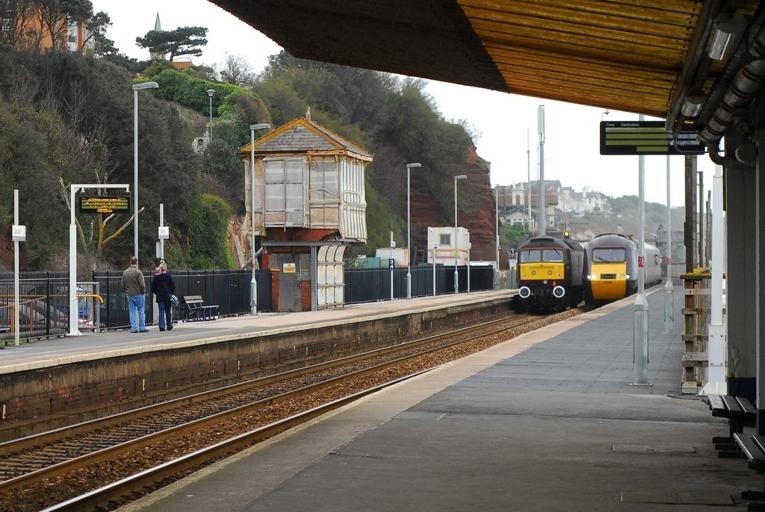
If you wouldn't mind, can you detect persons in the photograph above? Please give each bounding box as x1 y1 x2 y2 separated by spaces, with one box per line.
121 256 176 333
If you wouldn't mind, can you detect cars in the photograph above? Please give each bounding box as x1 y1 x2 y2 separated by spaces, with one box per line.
0 283 148 331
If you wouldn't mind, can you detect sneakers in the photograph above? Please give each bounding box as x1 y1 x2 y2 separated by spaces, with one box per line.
131 329 150 334
159 325 174 331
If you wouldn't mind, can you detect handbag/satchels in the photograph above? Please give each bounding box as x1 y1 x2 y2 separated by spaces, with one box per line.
170 295 180 308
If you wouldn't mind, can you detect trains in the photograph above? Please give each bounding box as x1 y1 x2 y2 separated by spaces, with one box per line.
516 232 662 311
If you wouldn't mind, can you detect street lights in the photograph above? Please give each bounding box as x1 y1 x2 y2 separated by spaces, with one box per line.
453 175 468 294
406 163 422 299
131 82 160 331
250 123 271 315
207 88 215 144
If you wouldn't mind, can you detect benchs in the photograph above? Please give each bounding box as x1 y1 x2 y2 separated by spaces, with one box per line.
710 393 764 511
183 295 220 320
